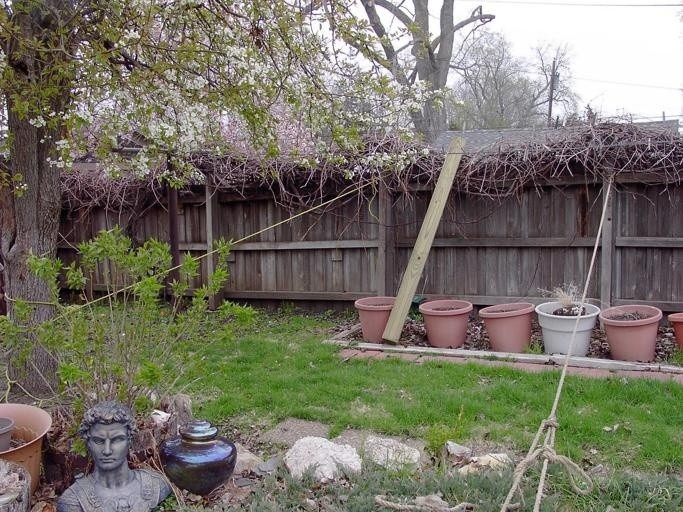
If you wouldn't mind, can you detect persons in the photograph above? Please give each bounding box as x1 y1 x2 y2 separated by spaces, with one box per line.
56 400 174 512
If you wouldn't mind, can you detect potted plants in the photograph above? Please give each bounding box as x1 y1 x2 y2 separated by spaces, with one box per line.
0 222 259 497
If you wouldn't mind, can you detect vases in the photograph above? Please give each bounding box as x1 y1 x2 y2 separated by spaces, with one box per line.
0 403 53 495
